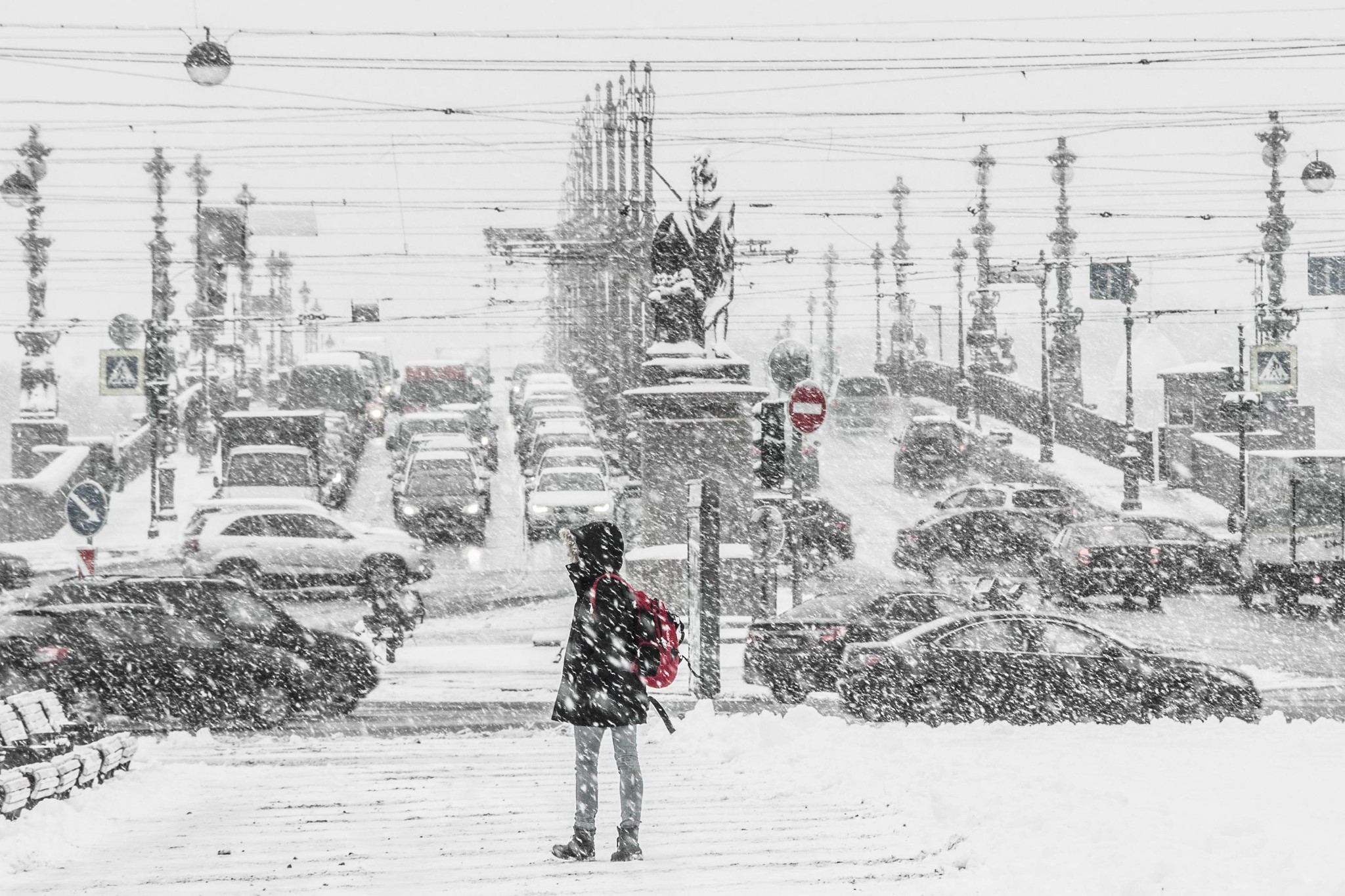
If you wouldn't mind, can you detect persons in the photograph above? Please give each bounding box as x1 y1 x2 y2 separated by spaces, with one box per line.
552 521 685 864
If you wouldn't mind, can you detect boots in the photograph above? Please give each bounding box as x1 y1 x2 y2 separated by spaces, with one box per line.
611 826 644 862
552 826 596 862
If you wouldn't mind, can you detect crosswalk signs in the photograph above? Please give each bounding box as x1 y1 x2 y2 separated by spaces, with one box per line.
1251 344 1299 395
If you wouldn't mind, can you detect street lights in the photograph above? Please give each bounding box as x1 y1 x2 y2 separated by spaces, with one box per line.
1256 109 1339 451
968 142 999 375
1045 136 1087 406
144 142 179 525
821 243 840 377
950 238 971 373
0 120 69 479
889 177 924 364
871 241 885 364
234 183 257 378
183 153 213 388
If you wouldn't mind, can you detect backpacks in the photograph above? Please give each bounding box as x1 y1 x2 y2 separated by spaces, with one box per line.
590 574 685 688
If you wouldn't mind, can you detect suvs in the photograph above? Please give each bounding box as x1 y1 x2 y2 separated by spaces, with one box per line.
177 496 435 596
26 572 384 702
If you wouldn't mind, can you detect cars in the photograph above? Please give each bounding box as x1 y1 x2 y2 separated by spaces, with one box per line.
890 415 1345 626
210 341 624 546
827 374 898 434
837 608 1261 725
744 586 985 706
0 602 316 732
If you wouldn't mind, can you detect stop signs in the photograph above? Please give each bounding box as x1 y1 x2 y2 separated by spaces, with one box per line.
788 382 826 434
